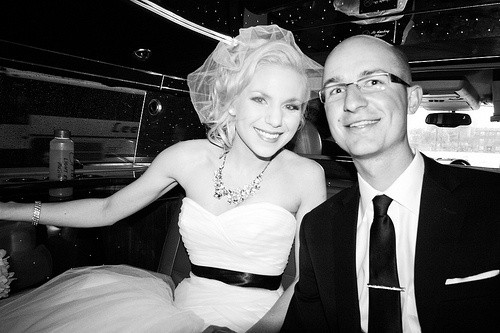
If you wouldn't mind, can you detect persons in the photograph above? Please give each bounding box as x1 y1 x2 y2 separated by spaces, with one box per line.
0 38 326 332
278 33 500 333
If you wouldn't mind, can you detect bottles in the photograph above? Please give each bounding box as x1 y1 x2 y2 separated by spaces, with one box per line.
49 129 75 197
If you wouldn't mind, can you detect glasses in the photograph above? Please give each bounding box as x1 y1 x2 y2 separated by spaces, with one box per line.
318 72 412 103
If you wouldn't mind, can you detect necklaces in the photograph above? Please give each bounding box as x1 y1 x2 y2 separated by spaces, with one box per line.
213 147 274 207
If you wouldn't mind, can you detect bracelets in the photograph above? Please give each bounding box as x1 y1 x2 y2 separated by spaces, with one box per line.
31 200 42 225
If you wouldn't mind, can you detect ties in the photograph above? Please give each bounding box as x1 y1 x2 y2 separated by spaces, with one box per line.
367 194 403 333
